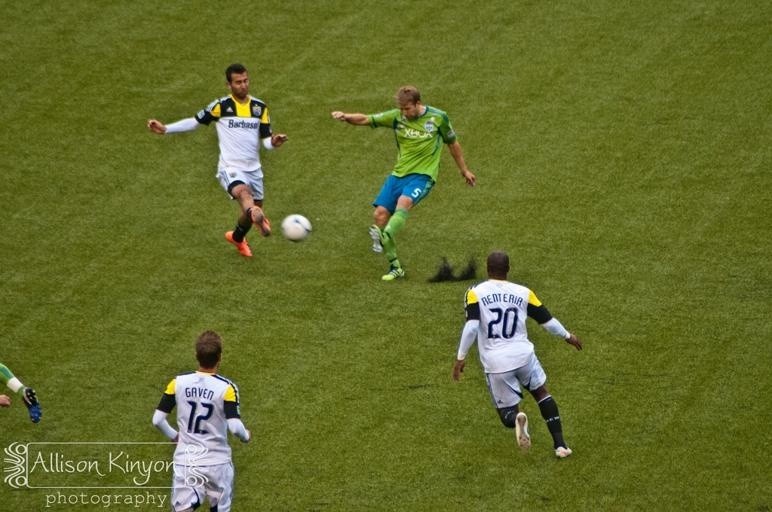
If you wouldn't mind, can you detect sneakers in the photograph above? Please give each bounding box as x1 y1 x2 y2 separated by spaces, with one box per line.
381 264 404 281
555 446 572 459
22 387 42 423
251 206 271 237
369 224 383 253
224 230 253 256
515 412 530 451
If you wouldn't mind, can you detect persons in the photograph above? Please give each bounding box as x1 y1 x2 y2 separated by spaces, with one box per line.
1 359 44 424
151 329 252 512
325 83 478 285
452 250 584 458
144 62 290 260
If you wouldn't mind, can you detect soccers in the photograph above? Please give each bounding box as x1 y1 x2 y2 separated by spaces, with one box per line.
280 214 311 242
